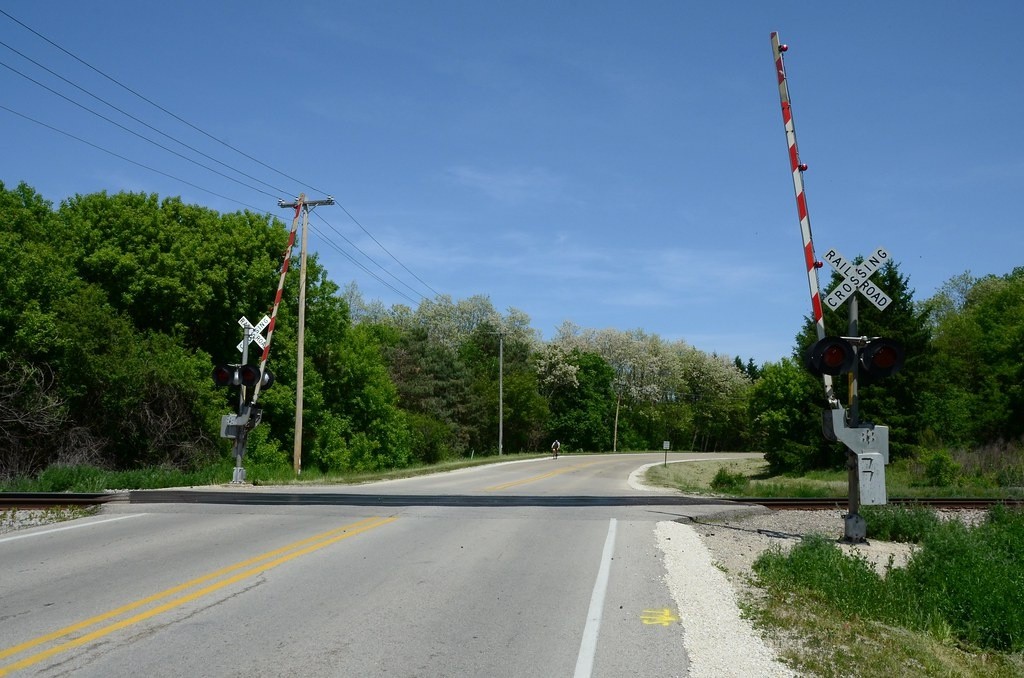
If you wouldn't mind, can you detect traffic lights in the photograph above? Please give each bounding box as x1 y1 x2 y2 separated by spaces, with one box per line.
237 363 261 387
804 336 854 374
862 337 909 378
211 363 233 387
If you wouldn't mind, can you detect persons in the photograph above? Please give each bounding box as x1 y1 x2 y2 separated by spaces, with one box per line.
551 440 560 456
